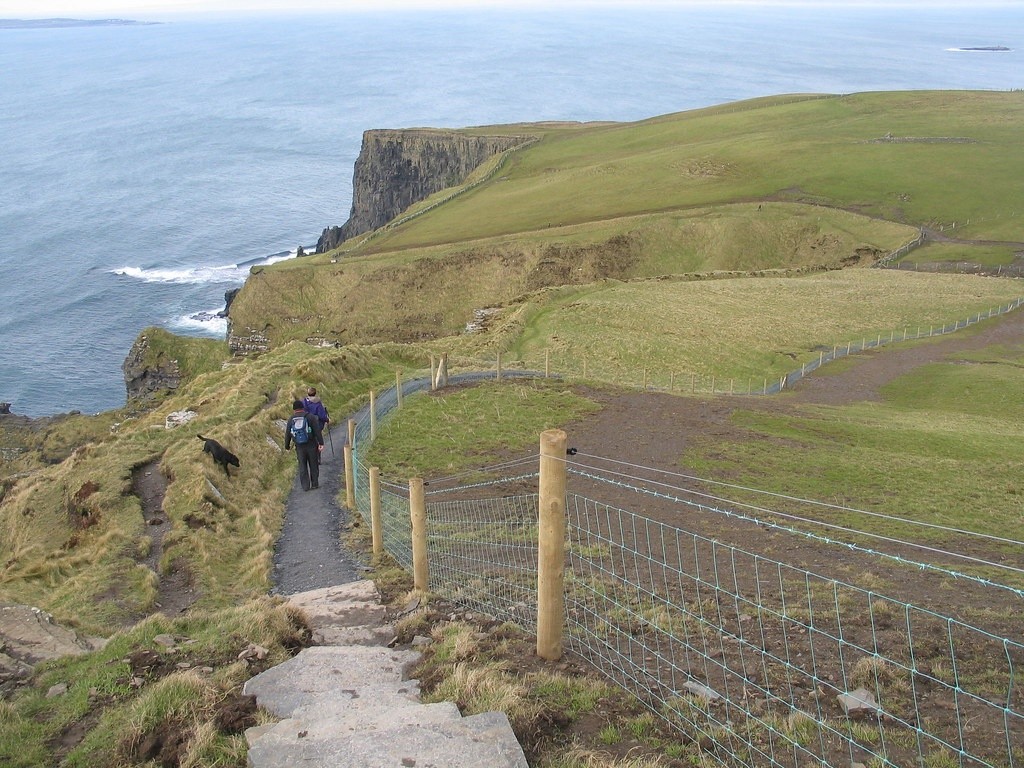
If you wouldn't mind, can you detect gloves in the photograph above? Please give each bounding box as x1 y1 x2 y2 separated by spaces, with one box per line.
285 445 290 450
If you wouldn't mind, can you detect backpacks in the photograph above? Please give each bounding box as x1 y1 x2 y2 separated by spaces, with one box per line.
290 412 312 444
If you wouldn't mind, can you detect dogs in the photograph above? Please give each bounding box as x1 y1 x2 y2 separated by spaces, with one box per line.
197 435 240 477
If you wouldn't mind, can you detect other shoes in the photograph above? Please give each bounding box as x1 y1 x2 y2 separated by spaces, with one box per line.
305 489 308 491
311 485 318 489
318 459 321 465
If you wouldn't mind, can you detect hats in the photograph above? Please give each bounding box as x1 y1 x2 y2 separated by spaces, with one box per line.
293 400 304 410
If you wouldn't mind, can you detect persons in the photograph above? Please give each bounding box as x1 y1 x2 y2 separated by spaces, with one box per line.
284 402 326 492
304 387 332 466
